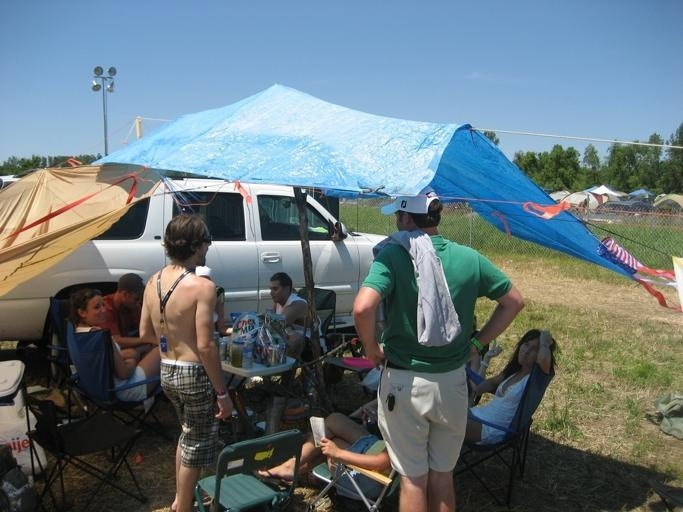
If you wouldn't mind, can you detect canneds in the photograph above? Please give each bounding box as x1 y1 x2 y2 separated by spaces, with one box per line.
278 343 286 364
267 344 278 366
218 341 226 361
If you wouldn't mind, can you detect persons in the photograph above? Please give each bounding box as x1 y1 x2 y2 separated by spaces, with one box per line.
462 326 559 448
467 318 502 408
97 272 151 346
68 286 162 401
351 184 525 511
139 215 235 511
254 413 402 498
266 271 327 394
201 273 249 390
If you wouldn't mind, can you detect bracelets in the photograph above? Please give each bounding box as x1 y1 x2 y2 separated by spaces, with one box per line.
214 388 229 397
539 342 550 348
469 336 484 351
480 360 488 368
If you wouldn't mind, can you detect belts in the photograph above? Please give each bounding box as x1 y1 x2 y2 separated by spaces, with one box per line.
383 360 413 371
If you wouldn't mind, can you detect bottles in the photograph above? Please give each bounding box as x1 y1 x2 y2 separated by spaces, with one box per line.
212 326 287 369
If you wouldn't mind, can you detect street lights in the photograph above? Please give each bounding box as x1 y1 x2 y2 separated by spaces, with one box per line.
91 64 118 159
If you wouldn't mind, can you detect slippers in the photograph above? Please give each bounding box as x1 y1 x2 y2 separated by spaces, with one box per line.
257 469 293 490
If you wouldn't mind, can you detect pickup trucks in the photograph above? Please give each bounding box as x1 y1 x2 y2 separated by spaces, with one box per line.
0 170 398 346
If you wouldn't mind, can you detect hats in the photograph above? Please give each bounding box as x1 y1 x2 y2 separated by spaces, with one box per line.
381 185 439 216
